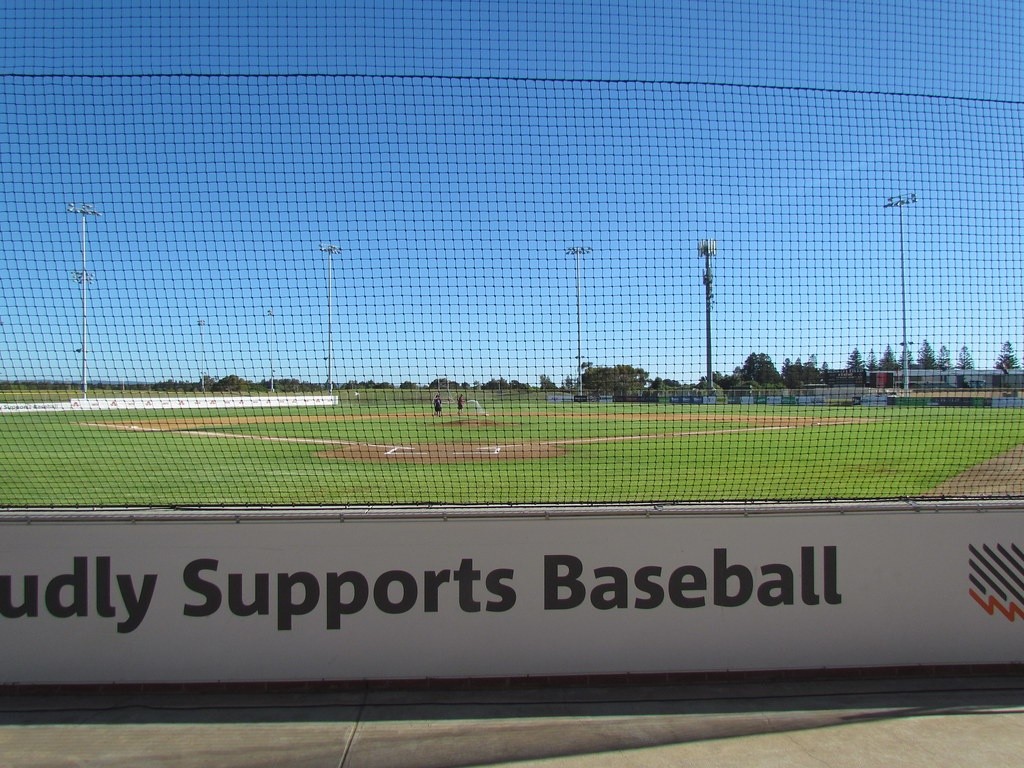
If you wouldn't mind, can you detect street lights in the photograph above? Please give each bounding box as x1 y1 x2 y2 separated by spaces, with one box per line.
266 308 275 393
695 238 717 389
883 193 919 390
566 245 594 397
319 243 343 395
197 319 206 392
63 202 103 399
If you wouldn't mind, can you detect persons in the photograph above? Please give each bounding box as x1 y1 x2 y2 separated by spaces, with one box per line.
434 395 443 417
458 394 464 413
